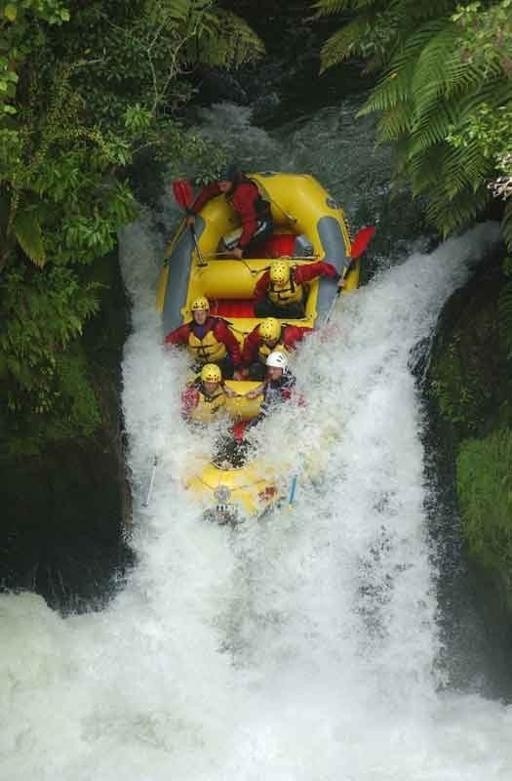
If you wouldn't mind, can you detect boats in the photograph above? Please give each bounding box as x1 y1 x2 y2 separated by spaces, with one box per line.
152 171 359 531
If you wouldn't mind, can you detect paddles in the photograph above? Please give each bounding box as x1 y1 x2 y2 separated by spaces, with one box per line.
323 226 375 324
173 176 208 266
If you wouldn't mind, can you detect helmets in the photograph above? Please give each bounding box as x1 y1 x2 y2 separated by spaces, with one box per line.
269 261 290 285
216 165 239 183
259 317 281 341
265 352 289 375
190 296 210 311
200 363 222 383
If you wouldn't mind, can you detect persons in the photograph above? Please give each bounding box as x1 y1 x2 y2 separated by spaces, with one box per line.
164 295 241 380
179 363 242 470
185 163 273 260
245 351 306 439
241 316 304 379
254 258 347 321
